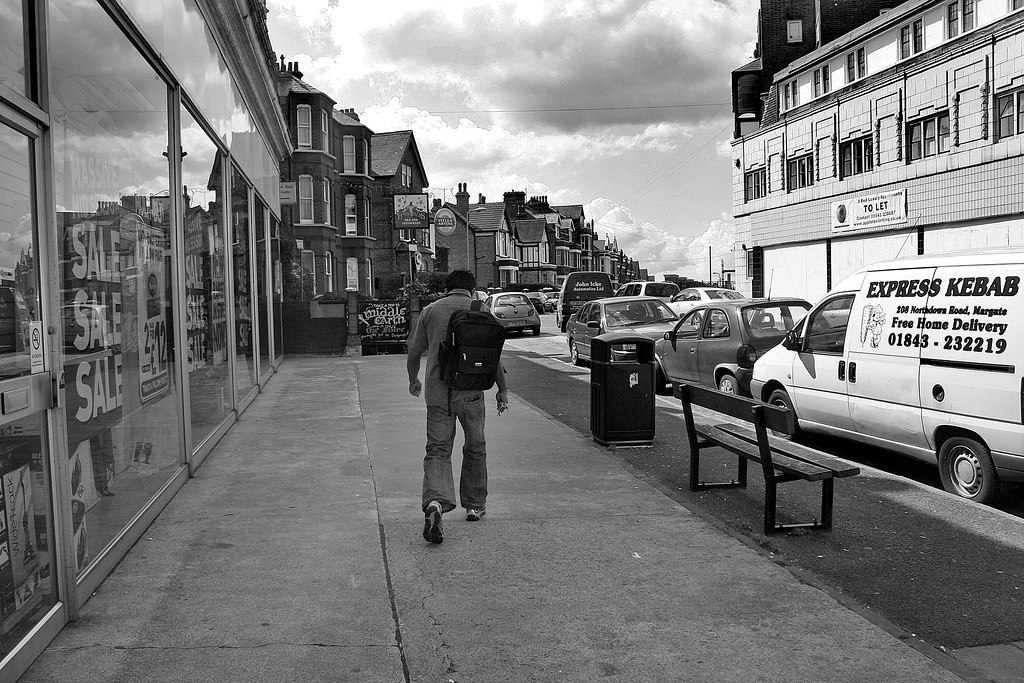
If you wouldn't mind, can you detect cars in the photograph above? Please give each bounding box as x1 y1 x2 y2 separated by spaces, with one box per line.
657 287 746 326
545 292 560 310
654 296 829 395
63 300 110 352
480 292 541 336
525 292 554 314
472 291 489 302
565 296 695 367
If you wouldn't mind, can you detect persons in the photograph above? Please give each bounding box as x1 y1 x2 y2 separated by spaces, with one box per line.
407 270 508 544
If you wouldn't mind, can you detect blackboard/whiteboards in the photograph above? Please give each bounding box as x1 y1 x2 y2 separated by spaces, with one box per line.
358 301 410 341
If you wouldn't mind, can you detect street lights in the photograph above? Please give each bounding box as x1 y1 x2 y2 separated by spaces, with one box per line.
713 273 720 281
467 207 486 271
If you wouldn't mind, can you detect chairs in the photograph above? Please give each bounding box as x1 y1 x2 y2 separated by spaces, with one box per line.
620 310 638 322
757 312 779 333
629 305 653 322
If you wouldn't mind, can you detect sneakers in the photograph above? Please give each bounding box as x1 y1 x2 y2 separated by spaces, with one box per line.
423 502 444 544
465 506 486 522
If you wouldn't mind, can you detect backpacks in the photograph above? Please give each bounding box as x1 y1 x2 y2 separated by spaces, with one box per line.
439 300 506 391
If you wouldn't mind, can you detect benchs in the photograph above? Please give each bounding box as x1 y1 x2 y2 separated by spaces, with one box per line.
671 375 861 535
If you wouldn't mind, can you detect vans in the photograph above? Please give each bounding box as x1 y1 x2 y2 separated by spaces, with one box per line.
0 286 31 348
554 271 613 333
749 245 1024 505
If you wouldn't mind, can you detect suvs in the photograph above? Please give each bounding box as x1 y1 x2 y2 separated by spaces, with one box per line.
613 279 681 318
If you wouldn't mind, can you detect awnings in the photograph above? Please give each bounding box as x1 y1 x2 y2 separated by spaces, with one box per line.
731 58 763 139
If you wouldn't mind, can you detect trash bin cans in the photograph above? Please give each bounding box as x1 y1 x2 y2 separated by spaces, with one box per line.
589 332 657 449
